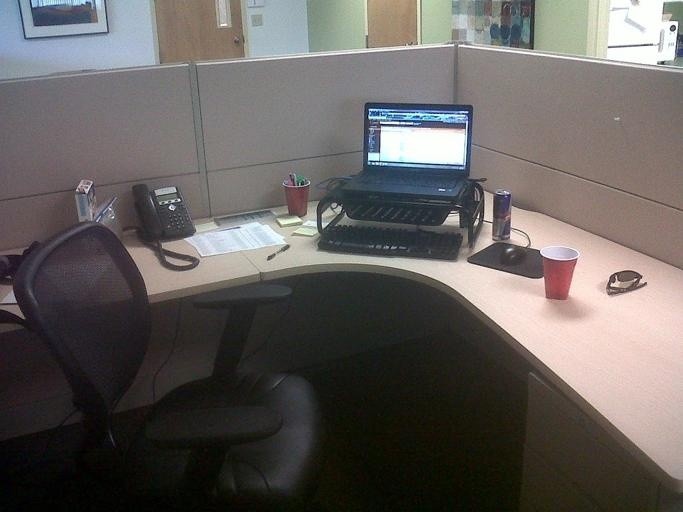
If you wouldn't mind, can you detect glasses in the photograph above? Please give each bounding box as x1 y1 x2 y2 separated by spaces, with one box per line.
606 270 647 295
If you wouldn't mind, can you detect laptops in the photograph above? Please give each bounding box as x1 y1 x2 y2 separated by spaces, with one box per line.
340 101 473 200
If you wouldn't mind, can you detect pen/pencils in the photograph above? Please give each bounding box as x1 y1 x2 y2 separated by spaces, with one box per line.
288 168 296 186
218 226 241 232
267 244 290 260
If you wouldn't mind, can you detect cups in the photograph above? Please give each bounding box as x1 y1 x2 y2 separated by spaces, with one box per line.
538 245 579 302
282 178 310 216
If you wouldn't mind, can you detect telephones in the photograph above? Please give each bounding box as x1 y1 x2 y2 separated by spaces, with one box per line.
132 183 197 239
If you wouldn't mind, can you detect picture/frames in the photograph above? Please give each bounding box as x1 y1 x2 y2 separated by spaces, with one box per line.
19 0 109 39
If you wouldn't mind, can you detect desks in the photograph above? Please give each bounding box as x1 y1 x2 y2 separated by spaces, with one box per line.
0 186 683 512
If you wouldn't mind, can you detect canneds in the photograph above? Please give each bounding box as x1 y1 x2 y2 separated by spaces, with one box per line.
492 189 511 241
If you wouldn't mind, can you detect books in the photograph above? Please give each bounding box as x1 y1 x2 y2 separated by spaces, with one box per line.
291 220 329 238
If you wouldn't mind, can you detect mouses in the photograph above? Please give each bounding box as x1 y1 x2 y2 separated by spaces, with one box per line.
500 242 527 265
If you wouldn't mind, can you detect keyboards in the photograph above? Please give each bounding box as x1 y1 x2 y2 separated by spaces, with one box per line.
316 224 462 260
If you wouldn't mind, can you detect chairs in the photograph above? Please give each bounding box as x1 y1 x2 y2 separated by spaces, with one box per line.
12 220 330 511
